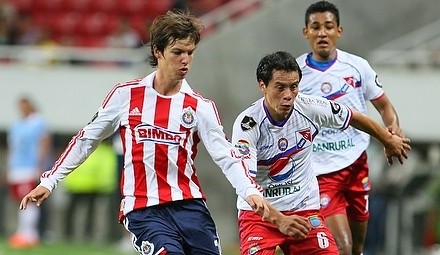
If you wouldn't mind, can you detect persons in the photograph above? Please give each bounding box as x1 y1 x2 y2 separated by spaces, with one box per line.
232 50 412 255
0 2 261 60
19 11 270 255
292 1 403 254
3 93 51 250
